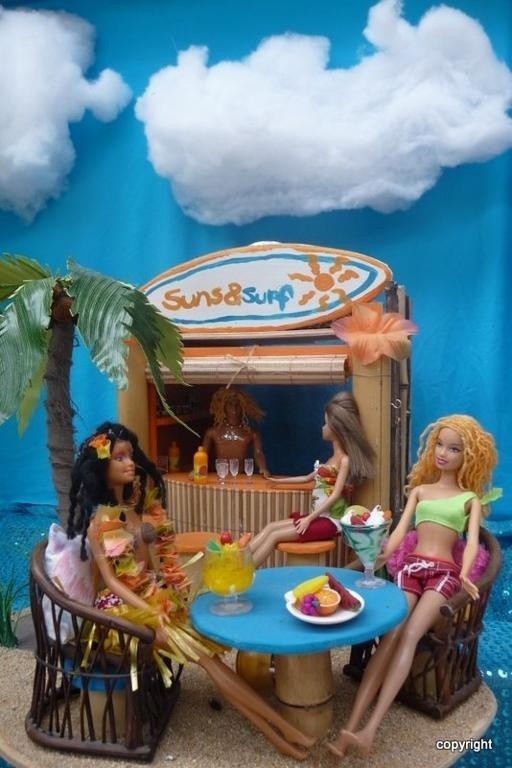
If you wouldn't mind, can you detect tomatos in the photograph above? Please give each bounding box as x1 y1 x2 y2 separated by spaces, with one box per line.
220 532 233 545
